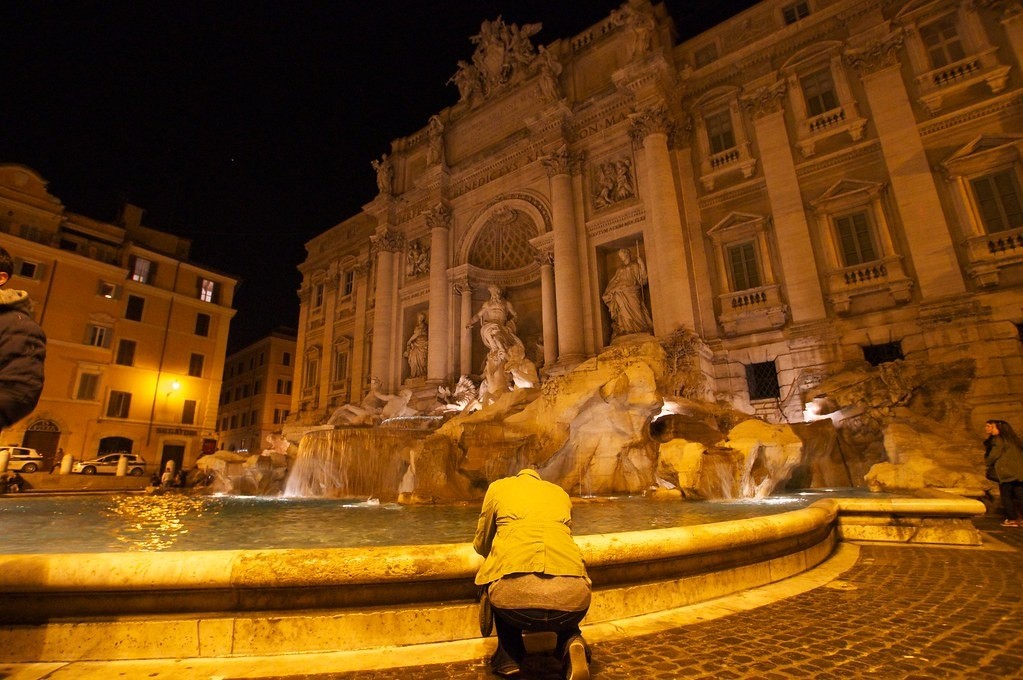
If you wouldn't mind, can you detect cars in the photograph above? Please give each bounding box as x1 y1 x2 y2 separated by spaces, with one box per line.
75 452 147 477
0 443 44 473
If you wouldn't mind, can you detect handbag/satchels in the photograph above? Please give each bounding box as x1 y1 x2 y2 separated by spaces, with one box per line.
479 594 493 637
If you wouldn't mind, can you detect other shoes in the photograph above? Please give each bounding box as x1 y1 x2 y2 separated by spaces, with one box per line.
565 637 590 680
497 662 520 675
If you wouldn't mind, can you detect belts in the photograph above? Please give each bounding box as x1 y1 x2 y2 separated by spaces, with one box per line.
512 609 566 618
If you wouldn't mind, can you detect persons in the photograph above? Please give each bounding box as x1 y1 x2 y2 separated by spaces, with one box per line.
371 154 392 194
49 447 63 473
530 44 565 100
0 469 26 495
601 248 652 331
592 159 636 206
328 379 416 424
426 114 446 166
465 351 538 412
466 284 524 363
148 468 188 488
983 420 1023 527
404 311 427 376
0 245 48 433
469 16 542 87
609 4 655 56
446 60 484 107
404 242 430 276
473 468 593 680
